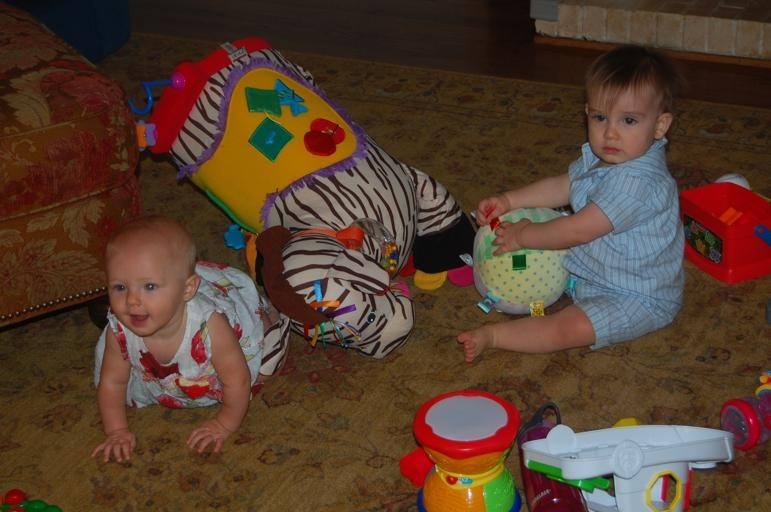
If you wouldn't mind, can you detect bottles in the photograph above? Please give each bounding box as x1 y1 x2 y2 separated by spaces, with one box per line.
517 402 589 511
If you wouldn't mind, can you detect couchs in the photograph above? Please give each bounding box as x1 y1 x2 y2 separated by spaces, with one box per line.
0 2 145 329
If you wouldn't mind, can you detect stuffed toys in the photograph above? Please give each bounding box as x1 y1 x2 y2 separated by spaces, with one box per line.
128 37 477 358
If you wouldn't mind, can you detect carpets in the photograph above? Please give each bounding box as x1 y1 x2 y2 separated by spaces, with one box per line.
0 29 771 512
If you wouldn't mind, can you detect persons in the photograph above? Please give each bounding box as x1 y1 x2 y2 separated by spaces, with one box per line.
92 214 281 463
458 43 685 363
